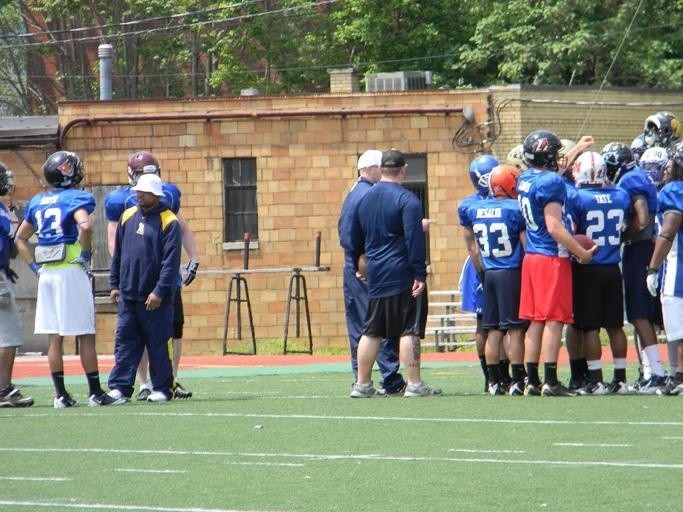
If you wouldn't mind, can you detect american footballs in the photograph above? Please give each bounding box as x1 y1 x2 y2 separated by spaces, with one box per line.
357 255 366 276
572 232 597 254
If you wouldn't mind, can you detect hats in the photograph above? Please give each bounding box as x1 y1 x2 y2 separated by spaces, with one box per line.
130 174 166 197
381 150 406 167
358 150 382 170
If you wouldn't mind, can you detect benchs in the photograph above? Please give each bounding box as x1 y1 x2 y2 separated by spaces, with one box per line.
421 289 477 353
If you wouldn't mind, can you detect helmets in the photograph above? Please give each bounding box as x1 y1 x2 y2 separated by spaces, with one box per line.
0 163 16 196
470 111 683 199
43 151 84 188
127 151 160 186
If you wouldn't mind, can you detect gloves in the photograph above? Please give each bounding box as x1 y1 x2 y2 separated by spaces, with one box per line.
68 251 92 275
646 268 659 297
29 260 42 277
0 281 11 312
182 260 199 286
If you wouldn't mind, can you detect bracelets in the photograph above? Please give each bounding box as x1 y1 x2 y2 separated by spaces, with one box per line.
29 260 41 275
79 249 90 263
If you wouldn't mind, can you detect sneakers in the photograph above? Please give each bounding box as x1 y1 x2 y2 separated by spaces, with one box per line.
485 373 683 396
136 383 192 402
88 389 128 407
351 381 442 398
0 389 34 407
54 394 80 408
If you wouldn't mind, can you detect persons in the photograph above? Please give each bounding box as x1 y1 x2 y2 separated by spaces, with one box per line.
105 173 176 404
13 150 131 407
338 149 437 395
104 152 200 400
459 110 683 395
350 150 443 399
0 161 35 409
